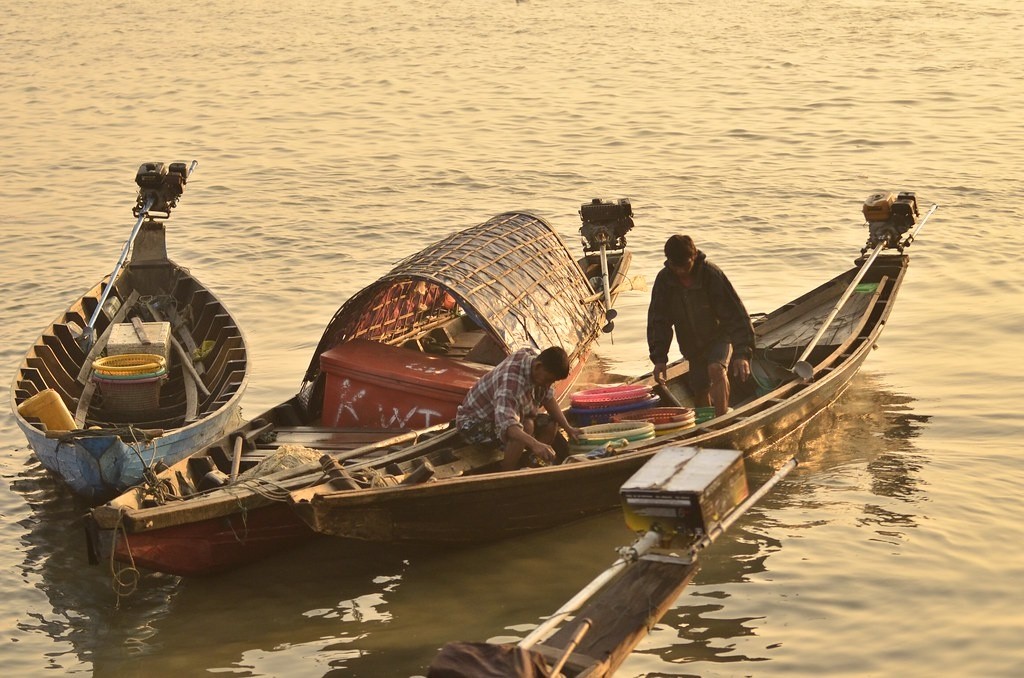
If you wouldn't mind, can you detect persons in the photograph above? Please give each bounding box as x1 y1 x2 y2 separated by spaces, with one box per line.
647 235 756 429
455 346 587 474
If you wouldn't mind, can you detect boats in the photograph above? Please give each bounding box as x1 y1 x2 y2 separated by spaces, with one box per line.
78 195 635 581
286 191 938 549
9 158 252 506
